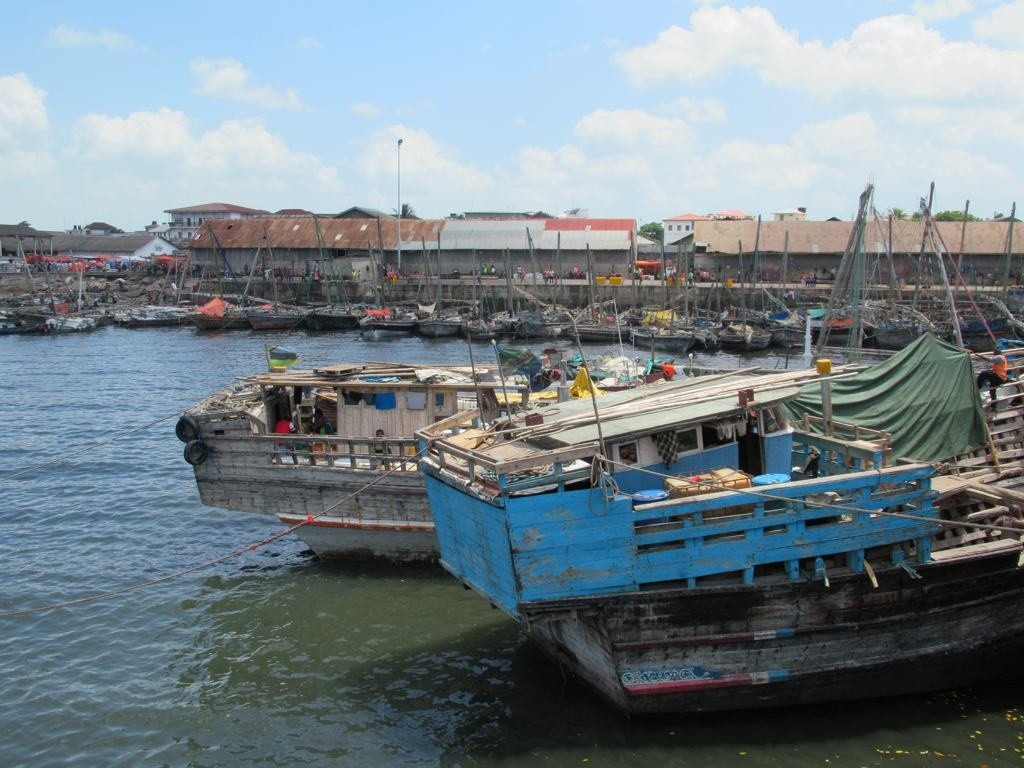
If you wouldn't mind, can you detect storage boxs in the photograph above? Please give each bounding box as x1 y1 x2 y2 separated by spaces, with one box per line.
664 468 751 518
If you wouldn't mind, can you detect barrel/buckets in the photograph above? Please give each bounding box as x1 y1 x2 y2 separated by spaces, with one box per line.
631 489 671 548
363 392 395 410
752 472 791 530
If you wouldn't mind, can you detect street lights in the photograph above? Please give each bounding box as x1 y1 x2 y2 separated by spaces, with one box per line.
398 139 403 270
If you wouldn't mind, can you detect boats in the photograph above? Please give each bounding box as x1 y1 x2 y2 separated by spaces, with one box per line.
175 363 793 557
413 293 1024 723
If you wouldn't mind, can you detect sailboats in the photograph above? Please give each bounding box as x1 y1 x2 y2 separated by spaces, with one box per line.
303 215 437 330
766 182 1024 348
616 214 773 353
182 222 313 332
105 254 194 326
0 234 108 334
461 227 632 342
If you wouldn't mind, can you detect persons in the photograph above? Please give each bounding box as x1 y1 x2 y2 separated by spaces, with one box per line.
275 408 334 450
975 345 1008 410
374 429 393 465
1010 367 1024 406
